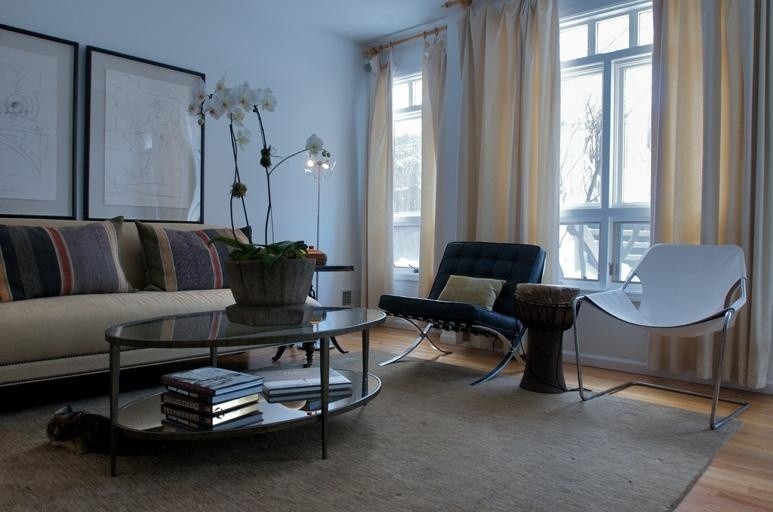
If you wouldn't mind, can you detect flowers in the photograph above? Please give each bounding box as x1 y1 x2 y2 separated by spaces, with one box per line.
185 76 324 261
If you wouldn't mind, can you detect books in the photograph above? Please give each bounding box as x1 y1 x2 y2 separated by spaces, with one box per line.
159 366 353 432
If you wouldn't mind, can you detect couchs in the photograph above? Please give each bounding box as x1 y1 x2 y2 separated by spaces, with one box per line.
0 223 323 421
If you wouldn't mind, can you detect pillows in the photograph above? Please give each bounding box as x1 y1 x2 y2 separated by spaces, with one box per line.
0 210 138 306
432 269 508 317
130 217 254 288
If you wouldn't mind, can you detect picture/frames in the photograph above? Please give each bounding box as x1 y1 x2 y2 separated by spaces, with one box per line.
0 24 78 220
82 41 206 226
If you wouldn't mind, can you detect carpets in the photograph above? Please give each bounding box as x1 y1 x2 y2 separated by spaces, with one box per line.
1 348 744 510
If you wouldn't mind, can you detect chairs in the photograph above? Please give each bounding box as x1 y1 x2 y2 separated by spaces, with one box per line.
378 240 545 383
570 241 750 430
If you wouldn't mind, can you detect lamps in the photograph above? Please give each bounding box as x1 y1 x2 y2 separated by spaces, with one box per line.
302 151 336 306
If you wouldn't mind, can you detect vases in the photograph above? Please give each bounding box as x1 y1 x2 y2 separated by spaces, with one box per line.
221 240 327 324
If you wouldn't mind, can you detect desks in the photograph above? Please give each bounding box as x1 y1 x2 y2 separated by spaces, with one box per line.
514 281 580 396
267 264 353 367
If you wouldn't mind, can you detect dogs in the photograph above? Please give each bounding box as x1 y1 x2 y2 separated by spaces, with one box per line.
45 406 255 471
